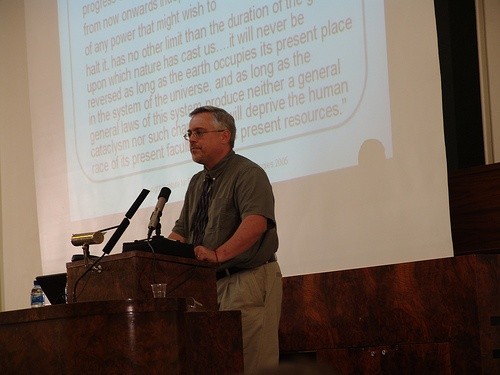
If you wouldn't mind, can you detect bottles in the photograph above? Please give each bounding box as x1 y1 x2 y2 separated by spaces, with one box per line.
31 285 44 308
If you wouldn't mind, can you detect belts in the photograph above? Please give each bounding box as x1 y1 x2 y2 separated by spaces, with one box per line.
215 263 249 280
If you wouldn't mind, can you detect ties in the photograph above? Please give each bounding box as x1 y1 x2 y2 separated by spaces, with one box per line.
191 174 215 247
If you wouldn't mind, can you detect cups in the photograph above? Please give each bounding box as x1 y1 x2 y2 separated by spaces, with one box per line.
151 284 167 299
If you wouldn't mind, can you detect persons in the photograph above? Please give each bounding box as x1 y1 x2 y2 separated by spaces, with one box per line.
168 105 283 375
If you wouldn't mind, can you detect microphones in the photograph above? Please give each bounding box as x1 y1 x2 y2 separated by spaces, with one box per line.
102 189 150 254
146 187 172 241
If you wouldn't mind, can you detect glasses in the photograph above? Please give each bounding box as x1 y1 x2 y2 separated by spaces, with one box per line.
184 130 224 140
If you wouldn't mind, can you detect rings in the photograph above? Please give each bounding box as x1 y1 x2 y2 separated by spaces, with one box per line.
198 256 199 259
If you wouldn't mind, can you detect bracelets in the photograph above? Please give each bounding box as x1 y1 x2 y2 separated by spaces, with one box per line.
214 249 219 262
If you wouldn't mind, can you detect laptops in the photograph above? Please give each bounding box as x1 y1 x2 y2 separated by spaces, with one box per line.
35 273 68 304
